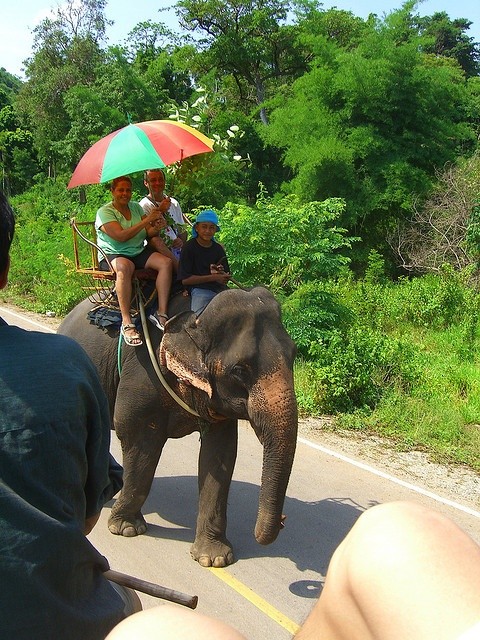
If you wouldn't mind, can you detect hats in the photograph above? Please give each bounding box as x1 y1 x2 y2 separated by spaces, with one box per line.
192 211 220 232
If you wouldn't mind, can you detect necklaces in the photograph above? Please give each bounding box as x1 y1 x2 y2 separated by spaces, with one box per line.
94 176 174 346
196 238 211 246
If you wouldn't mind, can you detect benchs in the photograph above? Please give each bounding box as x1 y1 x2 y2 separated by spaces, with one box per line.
70 220 156 317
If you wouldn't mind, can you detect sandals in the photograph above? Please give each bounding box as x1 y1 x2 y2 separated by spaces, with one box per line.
120 323 143 346
147 311 170 333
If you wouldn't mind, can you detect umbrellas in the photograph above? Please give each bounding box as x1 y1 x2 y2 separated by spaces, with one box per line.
66 118 217 227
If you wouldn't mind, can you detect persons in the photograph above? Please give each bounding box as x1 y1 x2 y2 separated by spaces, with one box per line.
101 495 480 640
138 169 192 278
174 209 232 315
0 188 141 639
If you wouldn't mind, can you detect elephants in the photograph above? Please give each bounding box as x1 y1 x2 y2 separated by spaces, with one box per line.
56 286 298 567
